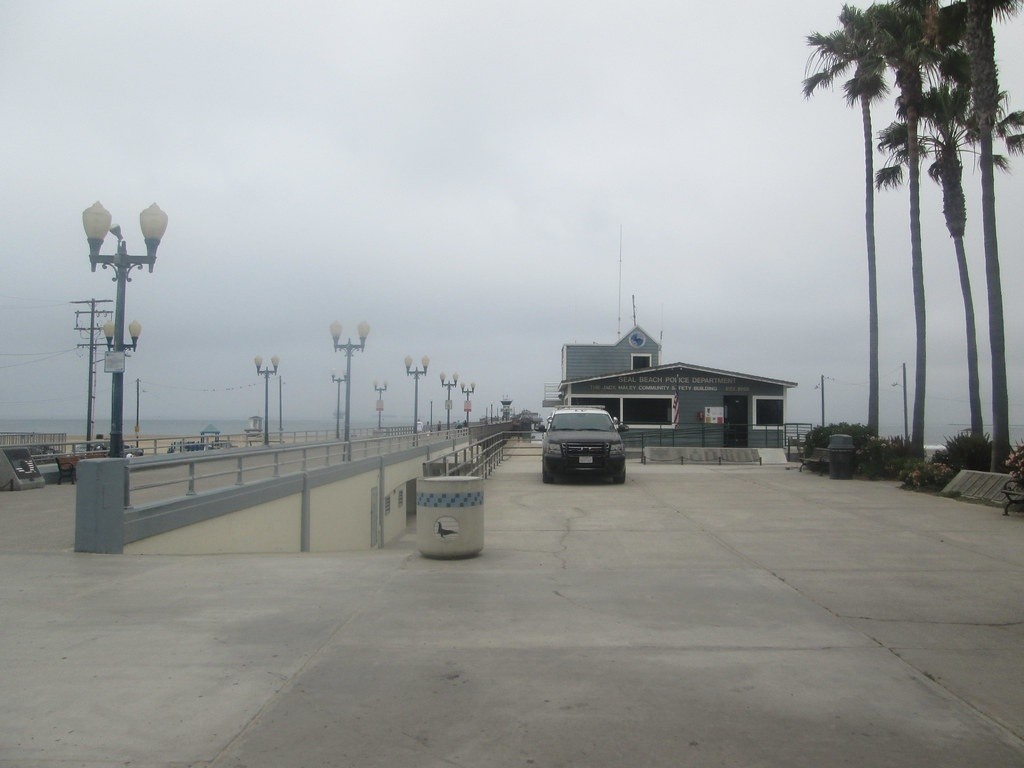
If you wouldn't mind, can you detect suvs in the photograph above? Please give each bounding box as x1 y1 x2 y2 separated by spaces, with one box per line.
539 403 626 486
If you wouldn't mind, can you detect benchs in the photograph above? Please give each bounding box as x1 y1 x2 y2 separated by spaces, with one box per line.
1001 479 1024 515
55 454 107 485
798 447 828 476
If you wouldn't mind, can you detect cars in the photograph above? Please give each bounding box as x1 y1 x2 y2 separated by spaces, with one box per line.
122 444 144 456
72 443 109 459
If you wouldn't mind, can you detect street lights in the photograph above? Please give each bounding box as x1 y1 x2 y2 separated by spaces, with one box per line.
81 200 169 455
462 381 476 435
329 320 370 462
490 400 494 423
890 362 909 443
372 375 390 430
406 353 429 447
439 370 460 440
813 374 825 430
255 354 282 446
